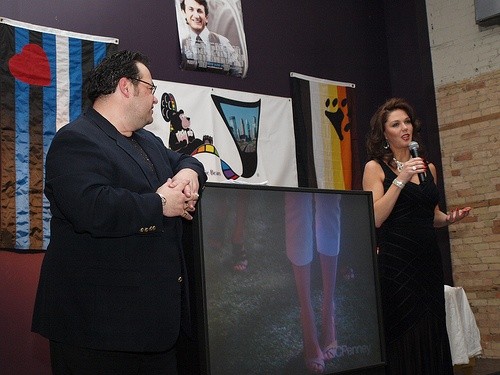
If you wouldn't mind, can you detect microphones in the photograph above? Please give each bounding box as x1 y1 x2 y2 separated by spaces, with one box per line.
408 141 426 186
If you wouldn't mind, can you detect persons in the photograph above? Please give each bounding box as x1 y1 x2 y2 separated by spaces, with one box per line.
32 50 208 375
285 192 343 374
181 0 241 76
362 98 471 375
208 188 249 273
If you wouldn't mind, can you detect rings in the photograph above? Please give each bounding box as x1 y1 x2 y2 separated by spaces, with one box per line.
186 203 189 210
412 166 416 170
182 210 188 217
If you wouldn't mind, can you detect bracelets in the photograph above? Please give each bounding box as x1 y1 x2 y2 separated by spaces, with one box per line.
158 193 167 206
392 179 405 189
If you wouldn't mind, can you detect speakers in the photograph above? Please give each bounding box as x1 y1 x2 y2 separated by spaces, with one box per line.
474 0 500 28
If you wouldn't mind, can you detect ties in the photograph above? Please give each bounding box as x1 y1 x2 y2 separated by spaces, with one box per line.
195 36 202 44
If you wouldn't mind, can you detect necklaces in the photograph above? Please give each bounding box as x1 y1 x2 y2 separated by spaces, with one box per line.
393 155 412 172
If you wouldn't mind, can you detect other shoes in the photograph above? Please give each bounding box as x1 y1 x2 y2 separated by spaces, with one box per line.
230 246 248 275
322 338 339 363
304 355 324 373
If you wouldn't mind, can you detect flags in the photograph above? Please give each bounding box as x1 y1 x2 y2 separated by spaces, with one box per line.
292 78 363 191
0 19 119 251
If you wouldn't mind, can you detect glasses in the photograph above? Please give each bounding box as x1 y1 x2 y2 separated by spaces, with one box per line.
129 78 157 94
